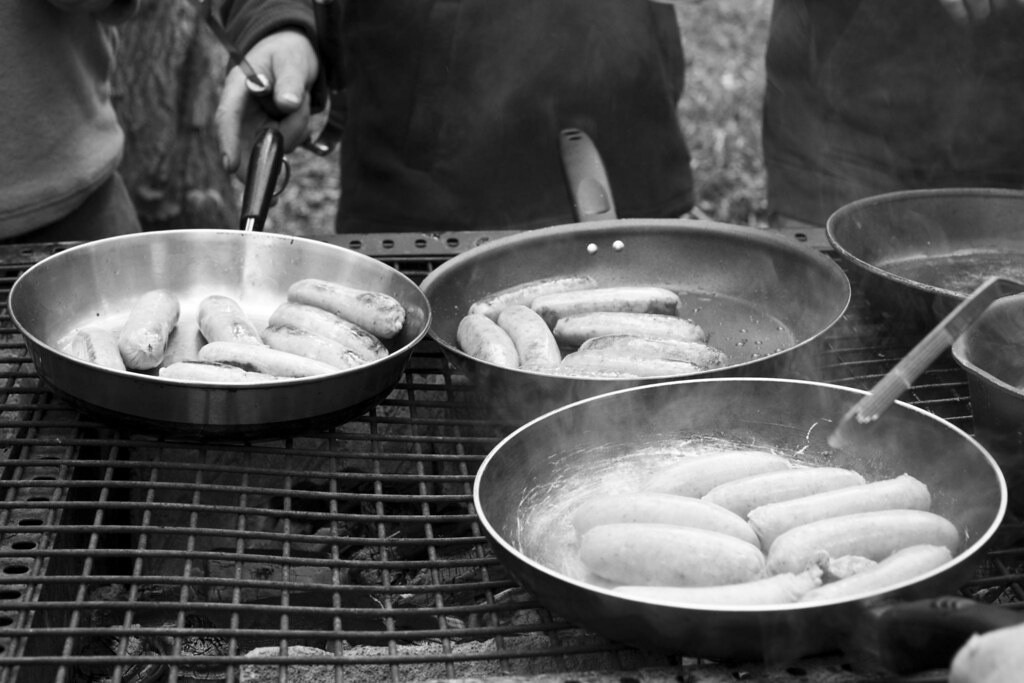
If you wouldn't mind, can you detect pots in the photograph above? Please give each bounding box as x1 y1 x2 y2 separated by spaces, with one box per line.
951 291 1024 450
474 277 1024 656
827 187 1023 330
418 128 851 418
8 127 431 424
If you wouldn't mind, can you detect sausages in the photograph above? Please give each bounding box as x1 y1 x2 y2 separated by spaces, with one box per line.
70 276 405 383
571 447 960 608
458 273 727 382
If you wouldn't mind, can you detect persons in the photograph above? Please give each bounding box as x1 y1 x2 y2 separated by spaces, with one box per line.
1 0 138 245
762 0 1024 226
212 0 695 234
